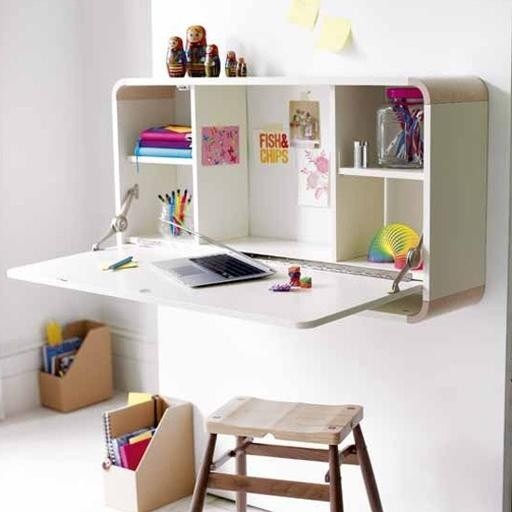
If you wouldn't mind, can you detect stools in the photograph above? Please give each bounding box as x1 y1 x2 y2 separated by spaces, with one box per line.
188 397 384 512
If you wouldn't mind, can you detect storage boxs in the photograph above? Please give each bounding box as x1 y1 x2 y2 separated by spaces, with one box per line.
98 395 195 512
39 320 113 413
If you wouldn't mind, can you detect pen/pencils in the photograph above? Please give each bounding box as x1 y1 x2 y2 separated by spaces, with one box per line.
102 256 133 271
158 189 191 238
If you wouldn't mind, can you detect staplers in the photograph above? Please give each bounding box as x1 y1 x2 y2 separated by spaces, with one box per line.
384 89 422 163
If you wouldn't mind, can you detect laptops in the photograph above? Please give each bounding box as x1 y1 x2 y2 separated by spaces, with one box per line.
151 217 276 289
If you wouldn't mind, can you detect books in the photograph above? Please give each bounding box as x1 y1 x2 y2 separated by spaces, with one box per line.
42 334 79 378
134 126 193 157
102 399 157 470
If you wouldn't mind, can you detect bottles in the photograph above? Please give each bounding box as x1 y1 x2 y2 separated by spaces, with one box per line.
375 87 424 169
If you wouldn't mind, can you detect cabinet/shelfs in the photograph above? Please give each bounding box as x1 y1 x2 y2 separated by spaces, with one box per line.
6 76 490 327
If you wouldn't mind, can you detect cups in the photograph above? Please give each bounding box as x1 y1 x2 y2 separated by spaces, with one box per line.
159 202 192 240
